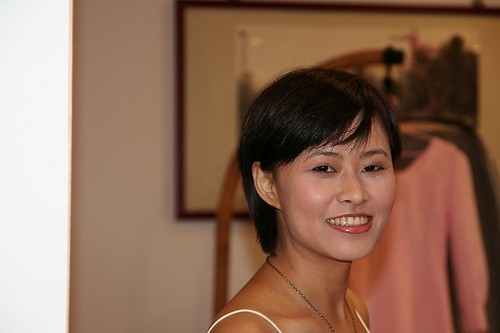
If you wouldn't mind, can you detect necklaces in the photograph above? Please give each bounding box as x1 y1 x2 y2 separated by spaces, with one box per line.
266 252 357 333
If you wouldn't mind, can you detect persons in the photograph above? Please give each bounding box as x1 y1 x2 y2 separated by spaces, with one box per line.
206 68 403 333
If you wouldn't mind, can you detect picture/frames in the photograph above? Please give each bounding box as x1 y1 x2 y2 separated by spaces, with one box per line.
172 0 500 222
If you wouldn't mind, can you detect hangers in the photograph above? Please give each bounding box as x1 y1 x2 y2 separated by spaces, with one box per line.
395 121 445 160
